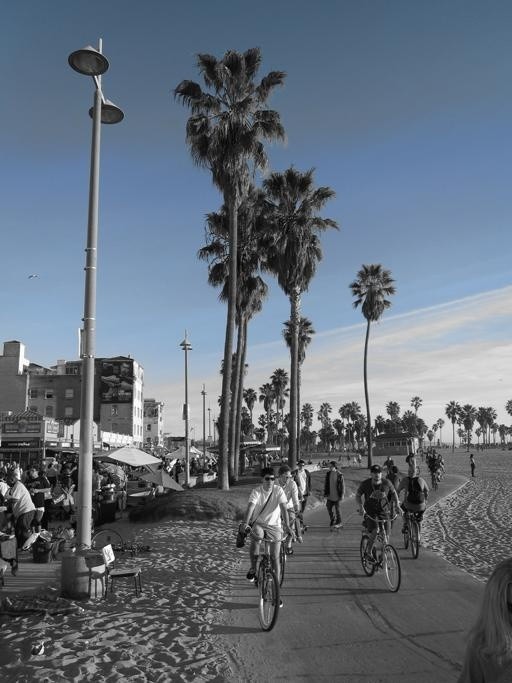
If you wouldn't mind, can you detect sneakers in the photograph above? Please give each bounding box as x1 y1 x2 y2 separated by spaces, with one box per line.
364 554 378 565
326 521 344 530
400 523 409 535
246 546 294 608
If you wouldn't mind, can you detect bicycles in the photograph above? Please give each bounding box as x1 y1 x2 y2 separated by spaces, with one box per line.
399 512 425 557
88 527 151 557
236 499 307 629
353 508 403 593
432 470 439 492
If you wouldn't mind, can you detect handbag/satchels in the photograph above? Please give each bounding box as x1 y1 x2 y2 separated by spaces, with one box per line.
233 523 247 547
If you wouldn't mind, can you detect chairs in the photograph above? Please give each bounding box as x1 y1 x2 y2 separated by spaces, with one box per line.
102 544 143 598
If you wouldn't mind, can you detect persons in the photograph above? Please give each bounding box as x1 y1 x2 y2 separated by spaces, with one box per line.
458 558 512 683
242 461 312 582
356 446 446 555
0 450 281 609
469 453 476 477
325 461 345 533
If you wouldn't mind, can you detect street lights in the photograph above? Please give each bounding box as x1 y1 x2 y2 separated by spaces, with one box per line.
176 326 192 488
63 31 124 603
200 381 217 456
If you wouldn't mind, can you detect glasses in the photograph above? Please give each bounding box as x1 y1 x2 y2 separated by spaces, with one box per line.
264 477 275 482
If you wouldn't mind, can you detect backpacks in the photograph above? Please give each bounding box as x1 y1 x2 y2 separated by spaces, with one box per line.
407 476 426 504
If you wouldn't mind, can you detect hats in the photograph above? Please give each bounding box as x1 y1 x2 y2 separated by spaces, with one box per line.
369 465 383 473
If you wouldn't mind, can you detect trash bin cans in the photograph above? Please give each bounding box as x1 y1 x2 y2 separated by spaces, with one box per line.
31 538 66 563
